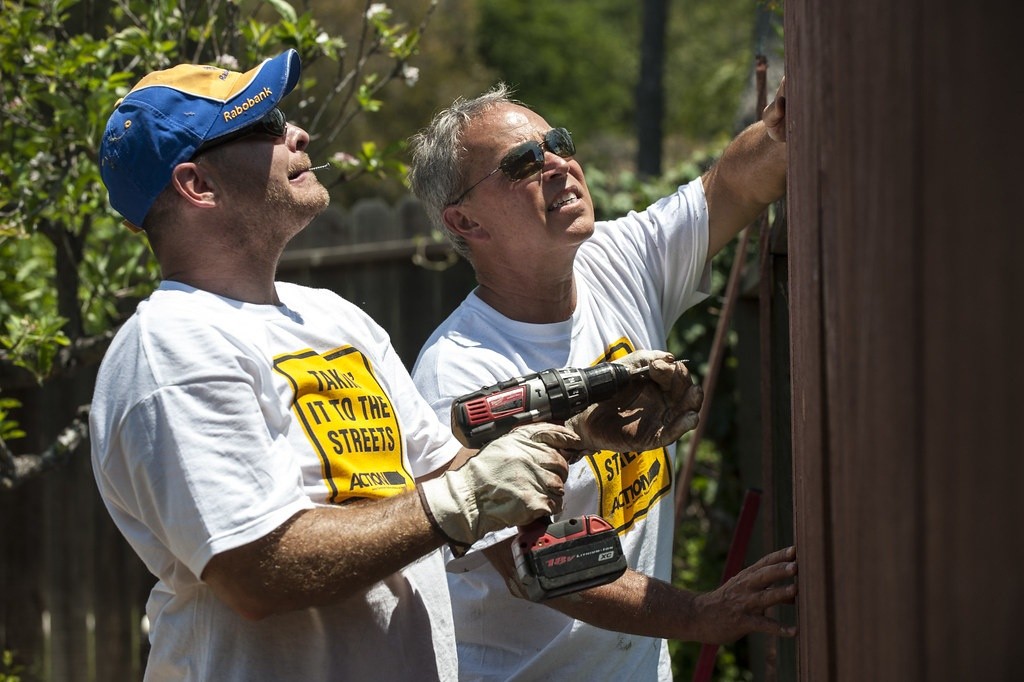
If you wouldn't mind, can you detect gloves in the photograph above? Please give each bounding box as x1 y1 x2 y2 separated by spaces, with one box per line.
415 421 586 558
565 348 704 465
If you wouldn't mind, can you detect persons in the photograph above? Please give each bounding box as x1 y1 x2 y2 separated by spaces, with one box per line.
89 47 704 682
412 74 797 682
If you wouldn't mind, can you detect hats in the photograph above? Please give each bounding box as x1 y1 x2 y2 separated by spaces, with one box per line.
99 48 301 233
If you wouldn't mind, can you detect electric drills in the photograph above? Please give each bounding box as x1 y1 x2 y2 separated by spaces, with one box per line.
449 357 691 604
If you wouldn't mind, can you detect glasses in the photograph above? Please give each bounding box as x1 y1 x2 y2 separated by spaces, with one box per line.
189 105 286 162
453 127 576 205
